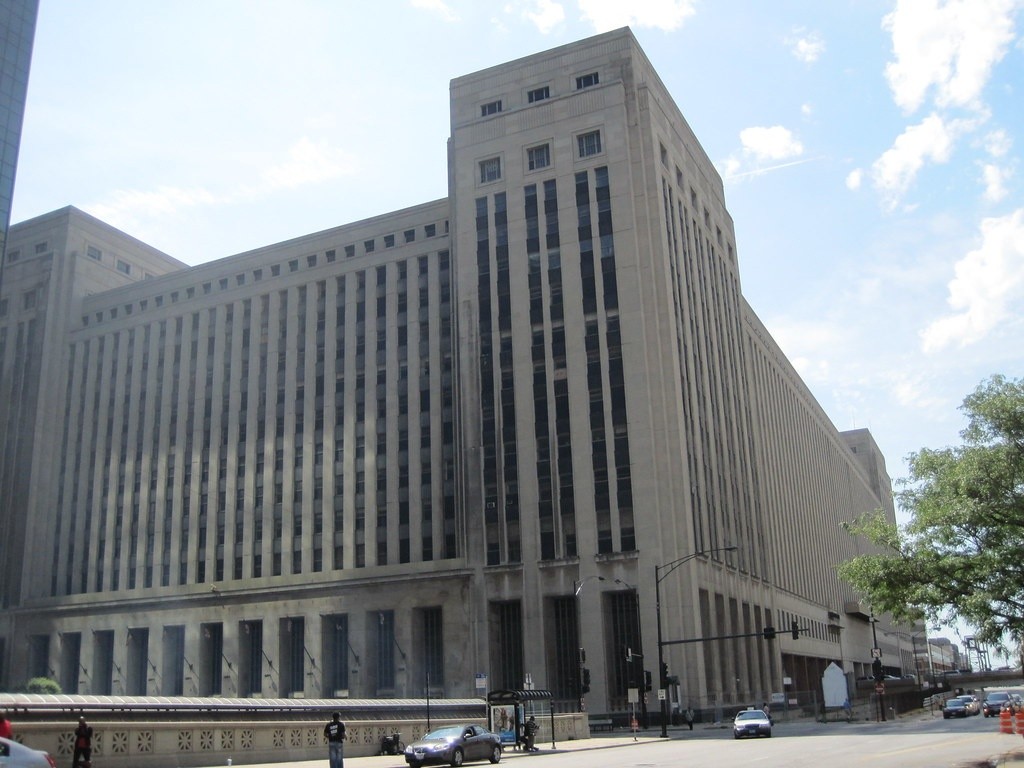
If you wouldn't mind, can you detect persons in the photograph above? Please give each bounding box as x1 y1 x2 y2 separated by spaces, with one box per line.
844 699 852 722
762 702 770 718
324 712 348 768
501 707 506 721
519 724 540 752
686 707 694 730
73 716 93 767
0 713 12 738
525 716 539 752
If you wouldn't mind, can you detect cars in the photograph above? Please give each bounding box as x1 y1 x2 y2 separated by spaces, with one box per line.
405 724 504 768
943 692 1023 719
733 710 772 738
0 735 55 768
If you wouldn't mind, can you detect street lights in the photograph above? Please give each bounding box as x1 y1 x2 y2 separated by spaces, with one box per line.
660 545 741 737
